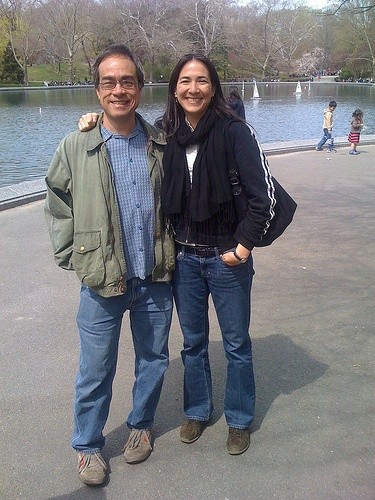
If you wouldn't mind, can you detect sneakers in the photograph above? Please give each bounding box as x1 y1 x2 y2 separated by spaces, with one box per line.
326 148 337 153
79 451 109 484
348 149 360 155
179 419 209 443
122 428 153 464
226 427 250 455
316 147 323 151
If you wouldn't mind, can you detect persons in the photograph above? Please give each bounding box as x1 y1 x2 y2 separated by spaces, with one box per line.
42 43 176 485
225 86 246 122
315 100 337 152
347 108 366 155
78 53 279 456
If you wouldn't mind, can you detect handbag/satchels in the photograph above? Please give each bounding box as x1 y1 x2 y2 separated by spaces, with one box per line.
224 118 297 247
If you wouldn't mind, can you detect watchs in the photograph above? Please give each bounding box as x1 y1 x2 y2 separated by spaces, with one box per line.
234 249 249 264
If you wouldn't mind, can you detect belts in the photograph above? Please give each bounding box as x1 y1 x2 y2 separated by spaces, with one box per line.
174 240 235 257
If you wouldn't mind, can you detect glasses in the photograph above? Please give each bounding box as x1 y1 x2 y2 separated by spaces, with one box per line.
96 79 140 90
